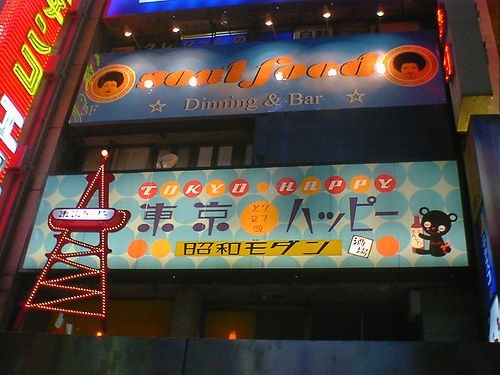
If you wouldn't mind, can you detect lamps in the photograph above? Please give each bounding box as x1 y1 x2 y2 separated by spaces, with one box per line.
322 5 332 37
123 22 142 50
376 4 386 33
264 12 279 39
220 11 235 43
172 16 190 48
